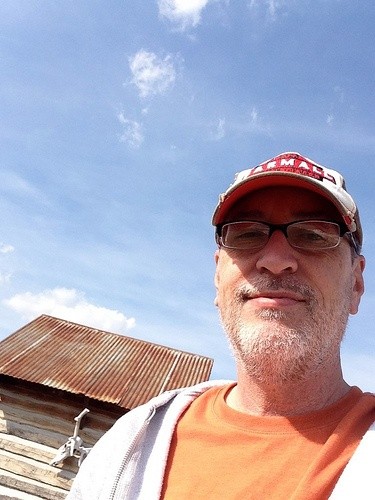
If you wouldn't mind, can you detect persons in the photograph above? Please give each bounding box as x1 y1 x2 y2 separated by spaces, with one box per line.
65 151 375 500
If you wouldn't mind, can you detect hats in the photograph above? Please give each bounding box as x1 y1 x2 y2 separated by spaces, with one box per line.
211 152 365 247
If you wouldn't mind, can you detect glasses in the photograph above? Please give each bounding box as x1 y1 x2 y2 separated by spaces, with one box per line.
214 218 360 260
